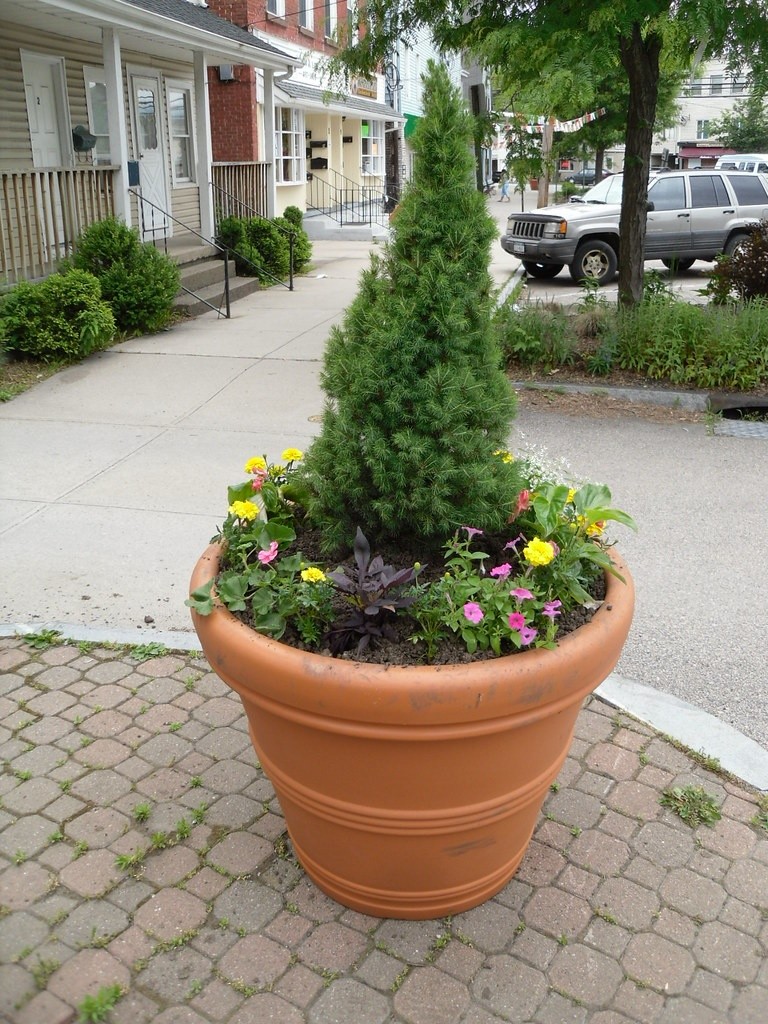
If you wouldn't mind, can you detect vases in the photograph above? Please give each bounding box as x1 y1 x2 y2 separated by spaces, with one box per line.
528 178 538 190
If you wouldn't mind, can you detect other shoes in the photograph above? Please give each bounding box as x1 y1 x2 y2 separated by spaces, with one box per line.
498 200 502 202
505 199 510 203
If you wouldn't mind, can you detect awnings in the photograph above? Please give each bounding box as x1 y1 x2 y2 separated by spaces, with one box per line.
678 147 737 159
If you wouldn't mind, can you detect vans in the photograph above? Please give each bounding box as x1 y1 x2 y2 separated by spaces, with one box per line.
714 153 768 173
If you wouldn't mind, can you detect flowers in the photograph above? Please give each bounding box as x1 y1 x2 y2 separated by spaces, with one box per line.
185 445 642 667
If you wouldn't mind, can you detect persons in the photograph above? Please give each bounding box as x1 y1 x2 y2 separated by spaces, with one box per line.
498 169 511 202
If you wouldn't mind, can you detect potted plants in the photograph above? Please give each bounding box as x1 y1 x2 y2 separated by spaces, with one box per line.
189 57 634 922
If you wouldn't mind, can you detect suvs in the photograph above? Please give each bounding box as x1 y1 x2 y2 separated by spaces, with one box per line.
499 168 768 287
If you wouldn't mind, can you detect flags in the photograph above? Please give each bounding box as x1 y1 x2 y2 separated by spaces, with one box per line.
554 108 607 133
491 111 545 133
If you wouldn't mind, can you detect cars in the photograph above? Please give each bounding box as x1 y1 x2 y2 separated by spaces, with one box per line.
565 168 613 186
